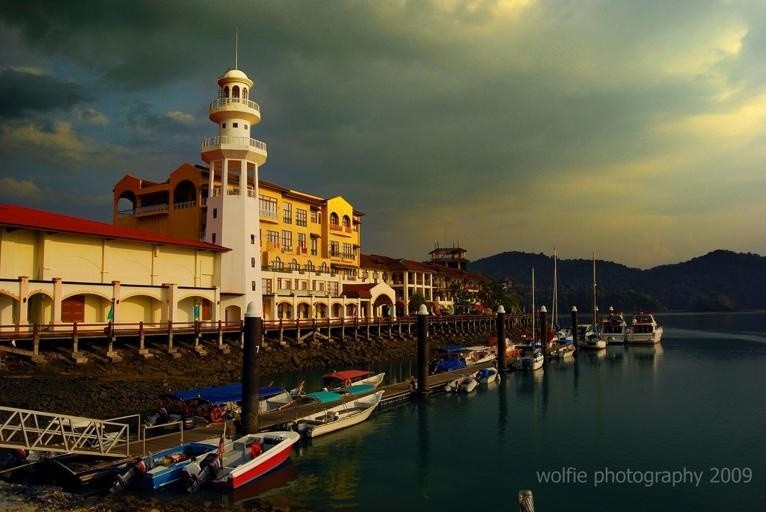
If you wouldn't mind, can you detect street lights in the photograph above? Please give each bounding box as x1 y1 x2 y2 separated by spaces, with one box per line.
242 302 262 432
493 304 509 371
414 300 432 403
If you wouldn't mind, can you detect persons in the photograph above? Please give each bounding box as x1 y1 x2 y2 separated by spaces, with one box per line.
156 406 170 426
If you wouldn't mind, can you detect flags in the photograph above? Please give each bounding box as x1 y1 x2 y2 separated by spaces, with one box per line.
216 434 227 460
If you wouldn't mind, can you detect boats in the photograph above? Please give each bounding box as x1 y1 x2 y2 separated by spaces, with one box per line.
24 418 122 461
137 435 236 496
600 304 628 345
445 391 476 402
293 391 389 438
464 345 496 367
515 351 544 372
224 460 302 502
427 346 467 373
473 366 498 383
486 248 603 358
606 344 626 370
303 416 379 449
475 374 501 400
517 367 544 394
626 344 664 376
548 357 576 368
445 378 479 394
587 349 607 360
319 367 386 398
625 309 663 345
185 430 304 491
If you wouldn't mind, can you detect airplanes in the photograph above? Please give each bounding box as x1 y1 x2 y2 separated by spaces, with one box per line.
145 378 305 430
